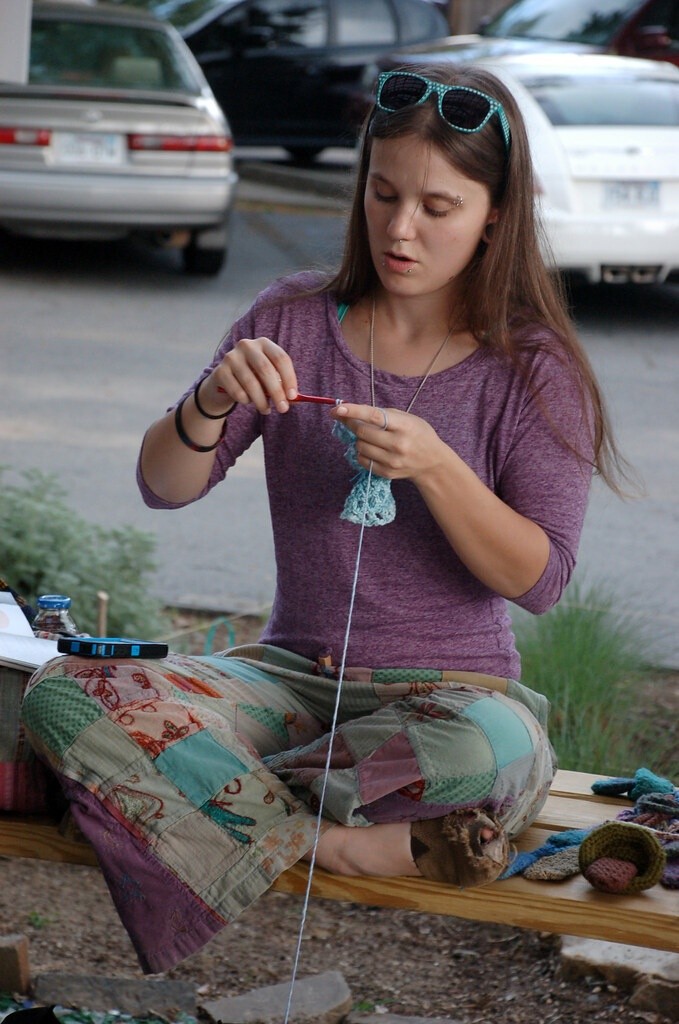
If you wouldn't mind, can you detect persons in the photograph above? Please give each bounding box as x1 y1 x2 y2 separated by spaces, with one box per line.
27 63 643 974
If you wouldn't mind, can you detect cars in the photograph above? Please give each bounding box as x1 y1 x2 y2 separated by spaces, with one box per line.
153 0 450 161
470 54 678 315
1 7 240 278
359 0 679 120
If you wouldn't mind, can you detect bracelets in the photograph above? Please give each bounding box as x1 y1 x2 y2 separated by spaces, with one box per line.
194 374 238 419
175 395 228 453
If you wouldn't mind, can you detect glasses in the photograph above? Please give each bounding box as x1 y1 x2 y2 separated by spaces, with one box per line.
376 71 511 151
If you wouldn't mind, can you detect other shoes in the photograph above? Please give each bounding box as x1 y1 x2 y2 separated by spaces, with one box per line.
409 804 508 888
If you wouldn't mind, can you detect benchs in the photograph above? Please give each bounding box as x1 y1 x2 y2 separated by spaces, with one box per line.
0 759 678 958
